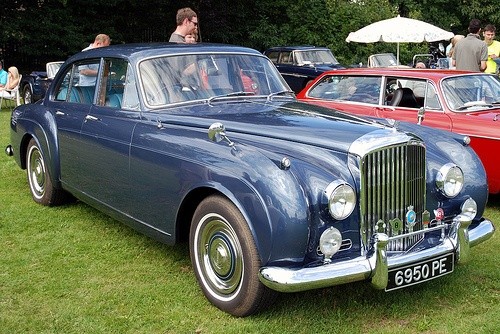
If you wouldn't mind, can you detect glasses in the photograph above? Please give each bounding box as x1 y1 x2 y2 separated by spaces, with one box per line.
188 18 198 26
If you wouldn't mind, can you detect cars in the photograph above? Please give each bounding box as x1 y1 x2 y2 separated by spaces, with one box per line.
295 68 500 198
263 46 455 96
5 42 495 320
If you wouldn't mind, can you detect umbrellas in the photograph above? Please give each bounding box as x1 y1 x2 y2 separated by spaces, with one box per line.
339 11 456 68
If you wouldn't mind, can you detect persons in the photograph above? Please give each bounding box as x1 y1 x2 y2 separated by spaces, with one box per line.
0 58 20 100
79 34 112 101
412 18 495 72
165 5 212 105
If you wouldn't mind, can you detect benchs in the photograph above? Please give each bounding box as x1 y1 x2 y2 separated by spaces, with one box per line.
56 85 125 105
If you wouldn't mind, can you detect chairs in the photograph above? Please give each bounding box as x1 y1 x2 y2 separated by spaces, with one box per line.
105 93 124 108
182 88 235 101
391 87 421 108
0 74 22 110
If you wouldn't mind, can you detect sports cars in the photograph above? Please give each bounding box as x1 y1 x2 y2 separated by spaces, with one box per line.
20 61 73 105
